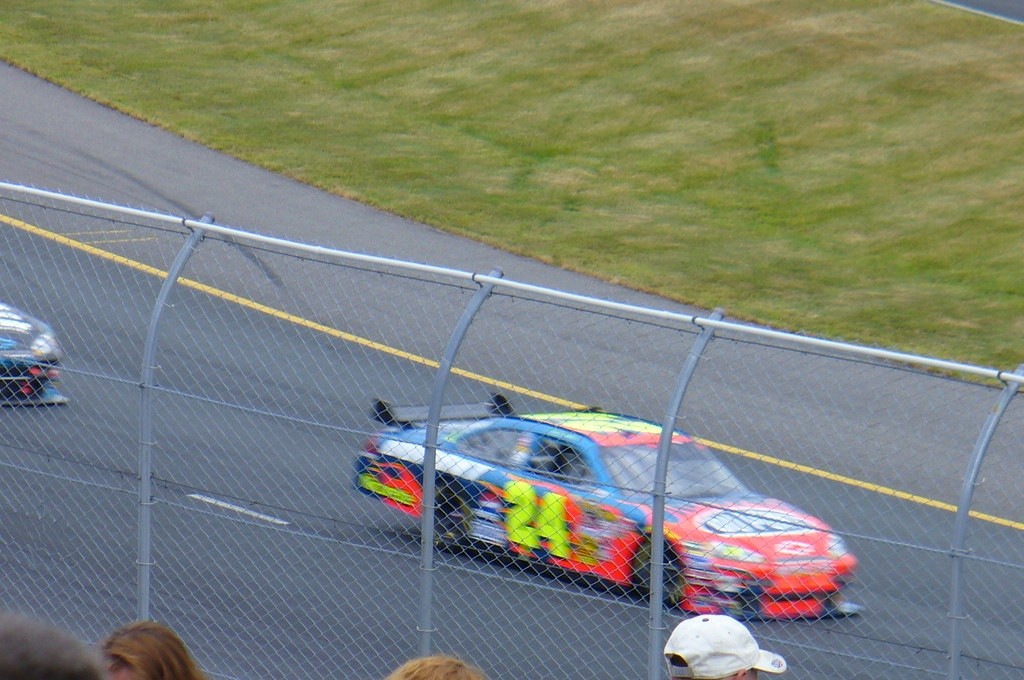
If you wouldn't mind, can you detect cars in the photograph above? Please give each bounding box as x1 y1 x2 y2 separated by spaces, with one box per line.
0 301 69 407
349 390 862 623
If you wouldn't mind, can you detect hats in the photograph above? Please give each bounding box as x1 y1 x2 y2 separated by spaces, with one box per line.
663 615 787 679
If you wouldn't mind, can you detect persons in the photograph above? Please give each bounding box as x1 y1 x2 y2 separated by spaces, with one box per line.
664 614 787 680
1 612 102 680
386 655 483 680
103 621 208 680
